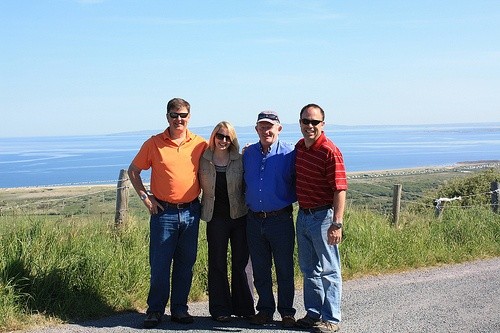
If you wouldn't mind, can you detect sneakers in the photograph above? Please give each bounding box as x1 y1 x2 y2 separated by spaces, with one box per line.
310 321 339 333
296 314 322 329
249 309 273 324
143 311 162 327
171 312 195 324
281 312 295 327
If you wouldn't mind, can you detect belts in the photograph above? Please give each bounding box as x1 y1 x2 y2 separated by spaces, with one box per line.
248 204 293 219
299 204 333 216
156 196 198 210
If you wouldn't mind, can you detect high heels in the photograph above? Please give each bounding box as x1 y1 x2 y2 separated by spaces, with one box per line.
209 312 231 321
235 309 255 318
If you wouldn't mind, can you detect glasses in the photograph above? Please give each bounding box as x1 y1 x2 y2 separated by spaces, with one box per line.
257 114 280 123
215 133 231 142
300 118 323 125
168 112 189 118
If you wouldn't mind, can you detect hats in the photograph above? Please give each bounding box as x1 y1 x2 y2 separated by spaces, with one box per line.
256 110 280 125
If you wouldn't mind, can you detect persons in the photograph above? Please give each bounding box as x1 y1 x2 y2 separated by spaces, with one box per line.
242 111 298 327
127 98 209 326
242 104 348 333
198 121 256 320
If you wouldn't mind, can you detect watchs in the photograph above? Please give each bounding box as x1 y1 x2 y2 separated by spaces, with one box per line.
141 194 148 200
332 222 343 228
